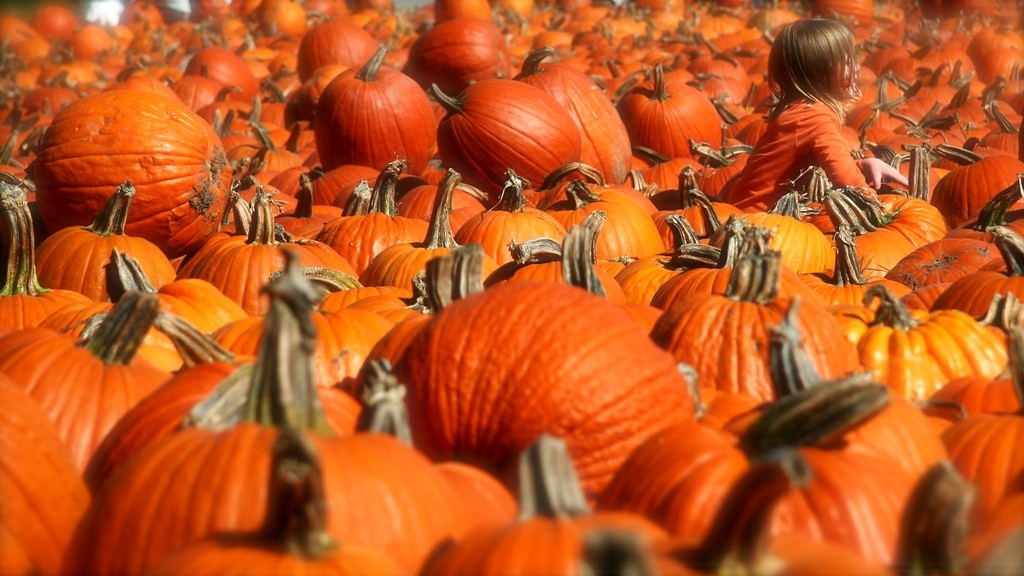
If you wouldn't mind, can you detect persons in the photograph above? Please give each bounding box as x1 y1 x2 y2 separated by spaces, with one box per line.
727 19 909 214
85 0 233 27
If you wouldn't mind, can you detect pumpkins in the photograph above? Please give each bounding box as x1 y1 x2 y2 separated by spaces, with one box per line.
0 0 1024 576
